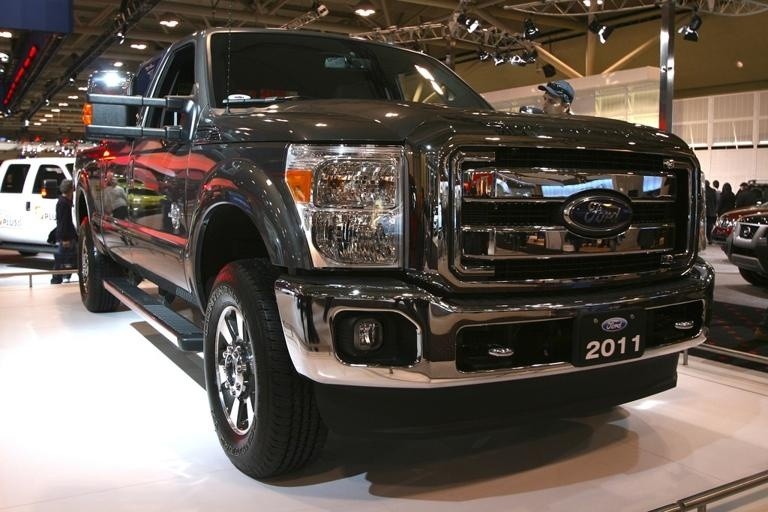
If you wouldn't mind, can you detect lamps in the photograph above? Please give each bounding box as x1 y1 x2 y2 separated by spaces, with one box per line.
677 6 703 43
456 15 557 79
587 14 615 45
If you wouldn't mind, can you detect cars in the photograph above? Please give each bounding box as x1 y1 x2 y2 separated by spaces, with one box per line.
710 201 768 286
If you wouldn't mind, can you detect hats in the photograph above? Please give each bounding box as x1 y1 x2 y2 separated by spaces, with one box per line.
538 80 575 102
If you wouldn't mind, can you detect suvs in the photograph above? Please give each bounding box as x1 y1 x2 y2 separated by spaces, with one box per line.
70 24 716 478
0 157 78 255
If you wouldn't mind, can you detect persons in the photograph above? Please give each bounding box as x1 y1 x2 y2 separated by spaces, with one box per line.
106 178 134 246
537 81 575 115
705 180 759 245
51 179 76 283
